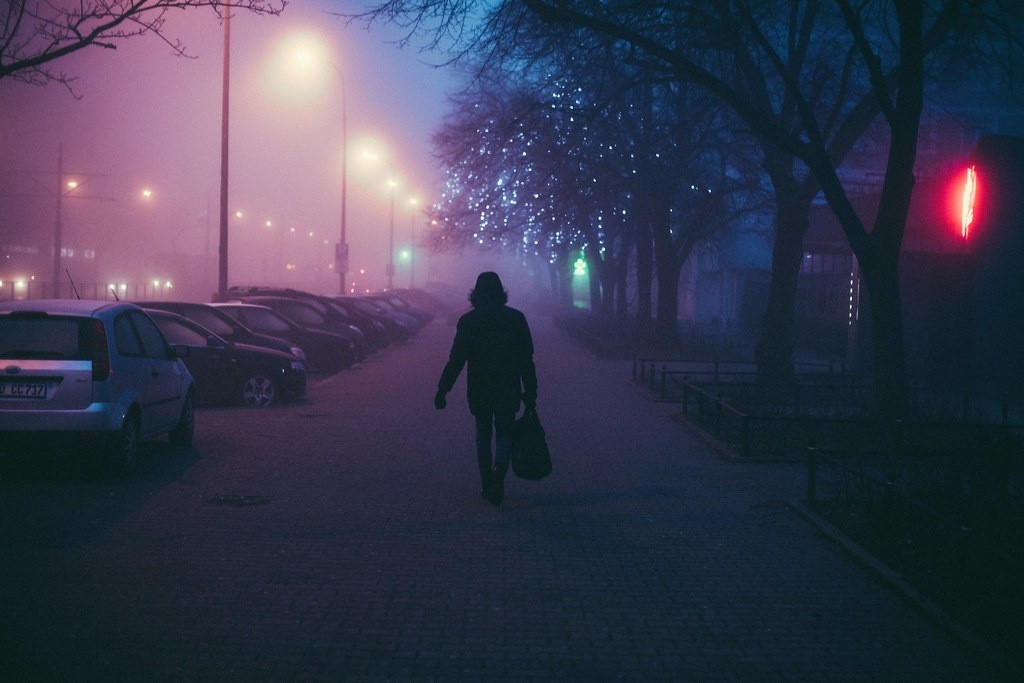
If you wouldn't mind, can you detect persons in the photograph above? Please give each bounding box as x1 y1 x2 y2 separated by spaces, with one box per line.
434 272 538 506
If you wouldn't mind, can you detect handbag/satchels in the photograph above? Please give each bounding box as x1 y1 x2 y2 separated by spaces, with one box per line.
510 406 552 481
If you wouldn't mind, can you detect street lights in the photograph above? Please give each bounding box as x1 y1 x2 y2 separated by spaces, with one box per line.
285 37 347 303
362 143 395 291
406 197 418 291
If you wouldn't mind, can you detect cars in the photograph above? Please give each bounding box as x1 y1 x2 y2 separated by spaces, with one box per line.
139 308 307 408
205 303 355 374
133 300 308 364
1 300 197 469
226 285 432 356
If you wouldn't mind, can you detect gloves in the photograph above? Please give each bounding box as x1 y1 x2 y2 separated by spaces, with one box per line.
435 389 447 409
522 391 537 407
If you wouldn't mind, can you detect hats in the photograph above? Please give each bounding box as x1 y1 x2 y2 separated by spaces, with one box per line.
474 272 503 297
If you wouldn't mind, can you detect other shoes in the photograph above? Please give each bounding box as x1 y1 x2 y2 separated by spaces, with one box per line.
480 485 503 505
